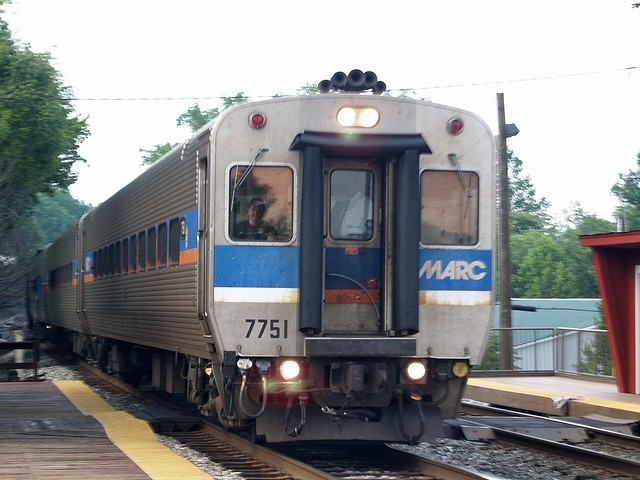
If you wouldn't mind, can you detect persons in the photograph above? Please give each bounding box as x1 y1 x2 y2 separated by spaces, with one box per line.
234 196 279 242
338 191 373 239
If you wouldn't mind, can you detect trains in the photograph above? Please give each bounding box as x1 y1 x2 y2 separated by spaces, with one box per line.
25 69 496 444
